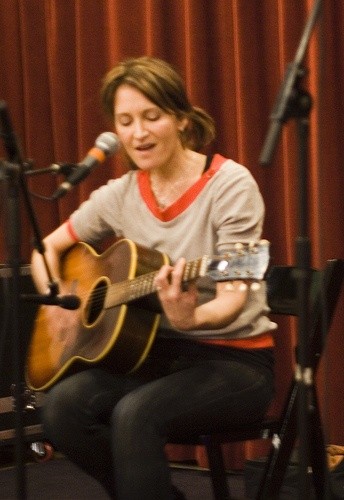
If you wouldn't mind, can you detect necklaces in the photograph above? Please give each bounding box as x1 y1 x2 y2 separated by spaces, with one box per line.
150 150 197 210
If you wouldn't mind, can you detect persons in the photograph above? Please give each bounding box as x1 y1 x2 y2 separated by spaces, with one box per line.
29 51 279 499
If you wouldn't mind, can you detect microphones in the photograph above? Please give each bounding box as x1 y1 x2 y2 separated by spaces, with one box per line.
53 131 121 201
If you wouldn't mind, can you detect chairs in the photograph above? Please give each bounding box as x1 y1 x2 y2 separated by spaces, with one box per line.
163 259 343 499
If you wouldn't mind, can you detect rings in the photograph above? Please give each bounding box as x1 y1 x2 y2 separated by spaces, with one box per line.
156 286 162 293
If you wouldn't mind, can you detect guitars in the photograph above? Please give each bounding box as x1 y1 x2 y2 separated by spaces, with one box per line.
26 239 271 392
